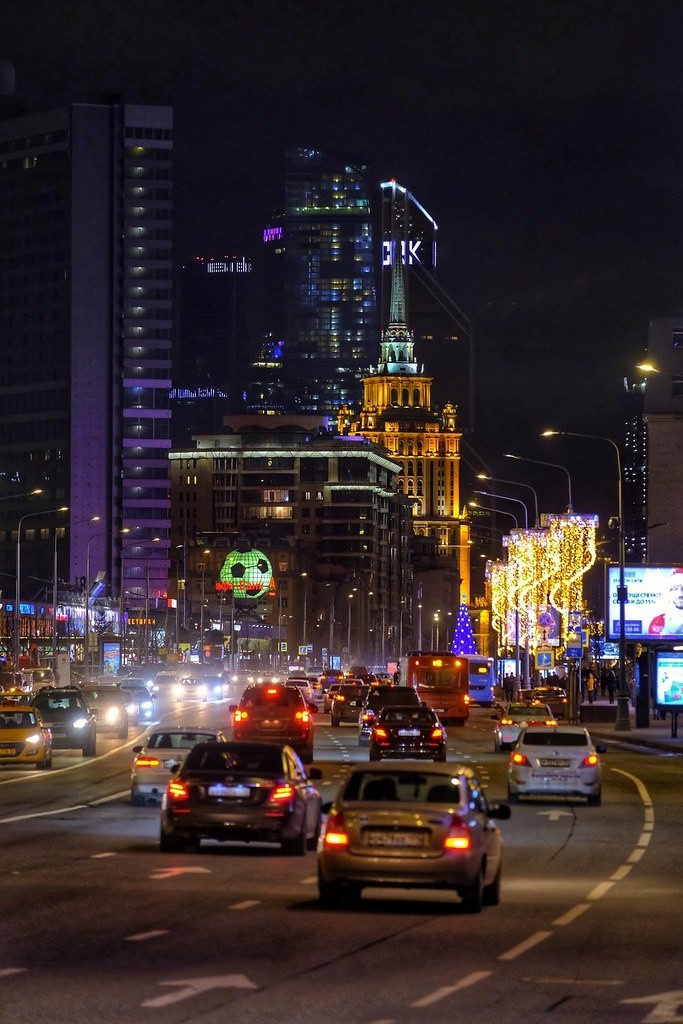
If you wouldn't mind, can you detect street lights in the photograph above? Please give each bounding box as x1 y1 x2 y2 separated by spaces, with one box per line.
144 543 183 666
476 474 544 688
542 431 632 731
230 562 263 655
118 536 161 664
200 557 234 662
52 515 100 669
278 571 307 672
331 591 354 670
504 452 580 726
13 506 70 673
84 527 130 679
303 582 330 671
474 490 531 690
218 567 250 645
176 550 211 654
469 501 522 692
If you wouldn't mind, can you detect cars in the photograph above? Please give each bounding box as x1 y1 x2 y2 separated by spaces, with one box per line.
315 761 512 913
499 724 609 807
158 739 325 857
490 702 560 754
130 725 229 806
0 706 53 770
0 644 497 763
524 685 569 720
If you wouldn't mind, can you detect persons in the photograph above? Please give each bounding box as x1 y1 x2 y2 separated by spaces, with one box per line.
69 699 77 707
22 714 31 724
648 567 683 635
652 706 666 720
381 777 398 799
498 669 636 707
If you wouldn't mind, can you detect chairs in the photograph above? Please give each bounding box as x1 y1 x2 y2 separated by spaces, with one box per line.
363 780 394 801
202 757 227 769
157 737 172 747
427 784 459 802
